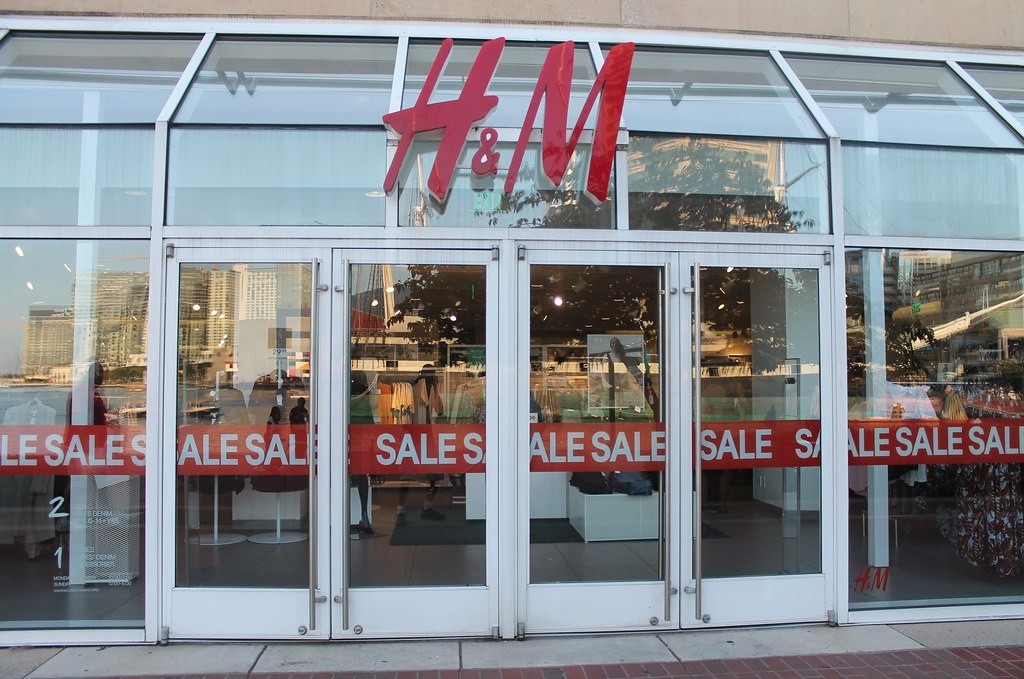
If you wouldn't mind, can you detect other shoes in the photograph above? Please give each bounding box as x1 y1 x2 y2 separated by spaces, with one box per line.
396 513 407 528
421 509 445 521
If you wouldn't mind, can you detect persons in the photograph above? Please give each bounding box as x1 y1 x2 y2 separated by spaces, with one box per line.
601 338 629 393
54 363 107 518
289 397 309 440
264 405 283 452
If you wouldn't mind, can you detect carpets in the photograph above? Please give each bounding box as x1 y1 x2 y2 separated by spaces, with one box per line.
389 500 732 546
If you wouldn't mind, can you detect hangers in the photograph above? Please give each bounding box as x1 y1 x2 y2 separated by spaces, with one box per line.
366 358 490 393
1 388 58 416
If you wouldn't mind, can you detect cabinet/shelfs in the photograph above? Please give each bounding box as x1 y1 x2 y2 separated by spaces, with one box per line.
465 472 698 543
186 475 373 526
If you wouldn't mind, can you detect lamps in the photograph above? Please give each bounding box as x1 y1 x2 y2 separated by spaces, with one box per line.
713 267 773 310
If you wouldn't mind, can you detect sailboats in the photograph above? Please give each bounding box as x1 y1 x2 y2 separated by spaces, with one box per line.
101 152 1024 422
